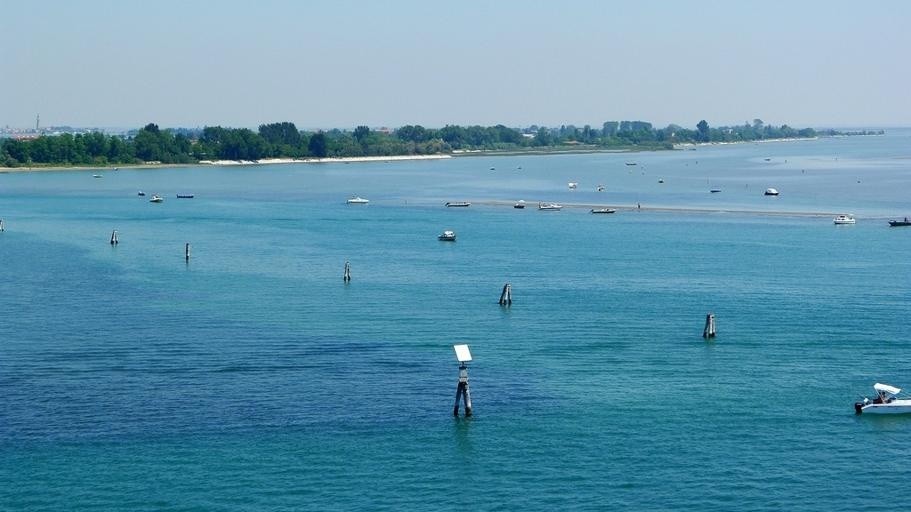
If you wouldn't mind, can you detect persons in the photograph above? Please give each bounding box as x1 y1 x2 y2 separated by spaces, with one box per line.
881 392 885 398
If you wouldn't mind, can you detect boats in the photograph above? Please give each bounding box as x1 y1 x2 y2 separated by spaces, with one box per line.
833 215 856 224
854 382 911 415
92 173 195 203
888 216 911 226
